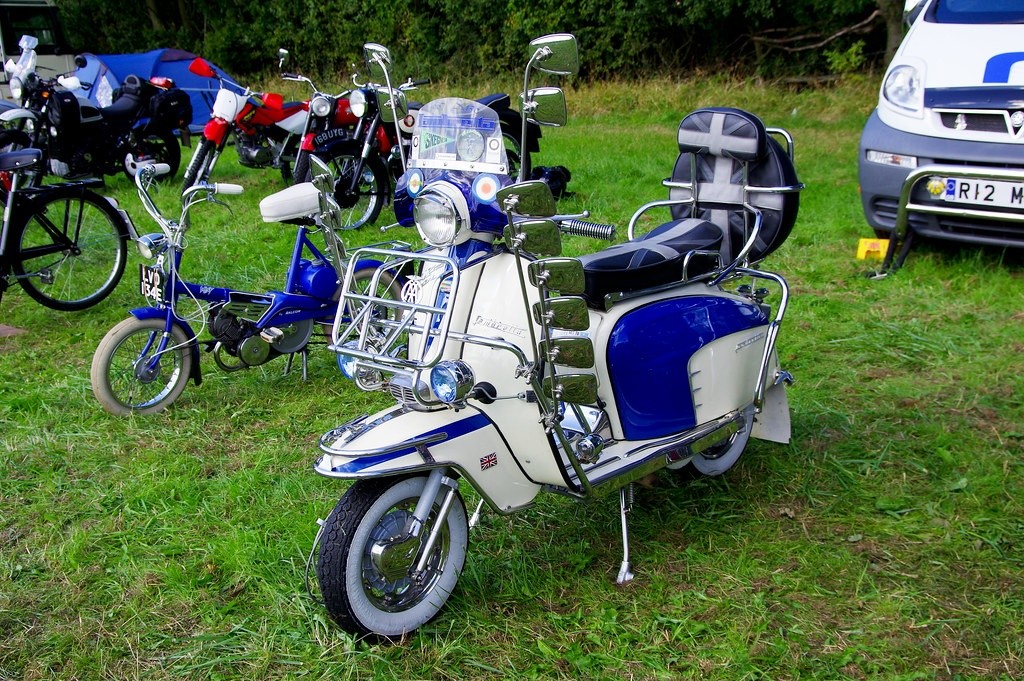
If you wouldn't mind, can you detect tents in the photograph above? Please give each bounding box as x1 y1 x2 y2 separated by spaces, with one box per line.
70 52 121 110
92 47 259 138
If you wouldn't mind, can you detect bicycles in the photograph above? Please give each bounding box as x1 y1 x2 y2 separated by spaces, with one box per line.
0 147 141 312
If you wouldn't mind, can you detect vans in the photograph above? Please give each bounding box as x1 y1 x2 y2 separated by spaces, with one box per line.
855 0 1024 253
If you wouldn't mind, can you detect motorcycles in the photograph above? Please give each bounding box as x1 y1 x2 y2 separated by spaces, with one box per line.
277 48 355 185
0 33 194 208
179 56 310 203
305 60 543 234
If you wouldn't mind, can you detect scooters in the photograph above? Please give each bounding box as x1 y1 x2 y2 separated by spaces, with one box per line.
90 152 417 418
310 32 808 650
